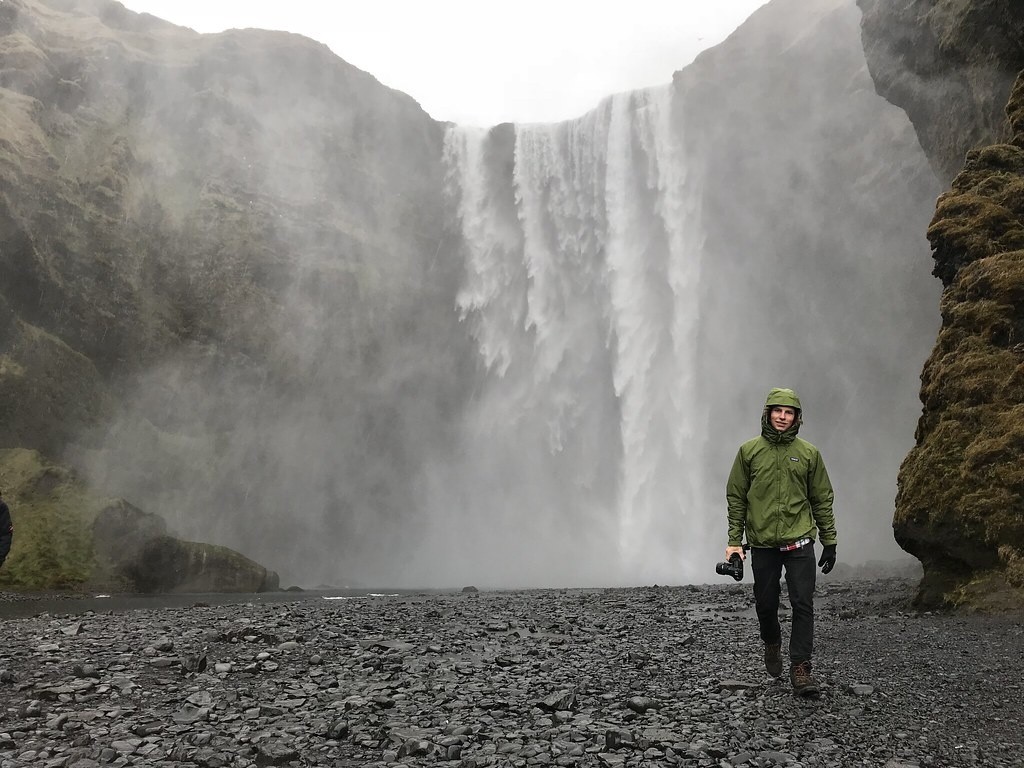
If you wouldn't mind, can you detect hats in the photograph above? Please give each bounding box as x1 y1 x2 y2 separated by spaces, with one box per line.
765 387 801 408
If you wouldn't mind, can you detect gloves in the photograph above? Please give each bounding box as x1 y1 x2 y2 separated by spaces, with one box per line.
818 544 836 574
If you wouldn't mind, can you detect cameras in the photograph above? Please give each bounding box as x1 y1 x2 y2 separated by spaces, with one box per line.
716 553 743 582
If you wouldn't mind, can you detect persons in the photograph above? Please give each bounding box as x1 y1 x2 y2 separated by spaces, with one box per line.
0 491 13 567
726 388 837 692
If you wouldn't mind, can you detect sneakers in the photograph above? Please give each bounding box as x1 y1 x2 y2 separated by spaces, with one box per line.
765 640 783 677
789 661 821 695
